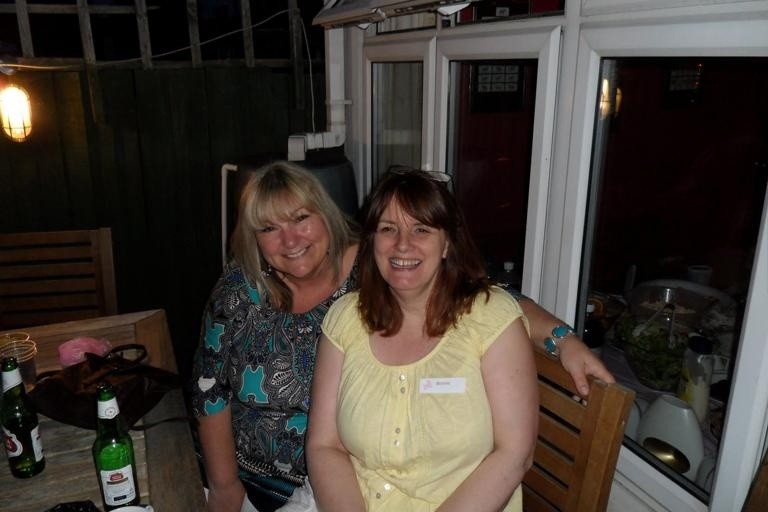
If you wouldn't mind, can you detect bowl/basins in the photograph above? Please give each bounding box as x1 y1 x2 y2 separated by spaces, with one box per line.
616 278 739 395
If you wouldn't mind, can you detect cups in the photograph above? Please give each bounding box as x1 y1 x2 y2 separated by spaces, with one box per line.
0 331 38 395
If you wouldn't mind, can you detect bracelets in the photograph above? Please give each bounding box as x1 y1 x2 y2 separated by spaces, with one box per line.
543 324 577 357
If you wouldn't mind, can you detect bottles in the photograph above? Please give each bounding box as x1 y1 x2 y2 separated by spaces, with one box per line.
497 261 518 291
675 336 715 425
0 356 47 480
91 380 141 511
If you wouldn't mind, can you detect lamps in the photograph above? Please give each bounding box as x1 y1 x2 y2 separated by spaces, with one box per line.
0 82 35 146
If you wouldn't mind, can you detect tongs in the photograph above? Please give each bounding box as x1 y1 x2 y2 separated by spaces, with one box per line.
629 301 678 352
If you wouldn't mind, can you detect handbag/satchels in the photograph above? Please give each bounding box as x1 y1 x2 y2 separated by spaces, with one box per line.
21 339 180 439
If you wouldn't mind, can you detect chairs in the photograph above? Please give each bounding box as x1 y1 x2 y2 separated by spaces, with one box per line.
520 352 635 510
2 227 118 332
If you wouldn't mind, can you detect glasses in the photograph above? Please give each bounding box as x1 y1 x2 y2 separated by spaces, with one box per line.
388 164 456 198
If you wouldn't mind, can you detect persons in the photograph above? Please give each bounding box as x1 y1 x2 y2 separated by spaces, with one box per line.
272 166 542 512
186 161 617 512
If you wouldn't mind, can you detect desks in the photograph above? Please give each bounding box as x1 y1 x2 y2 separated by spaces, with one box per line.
3 308 204 511
